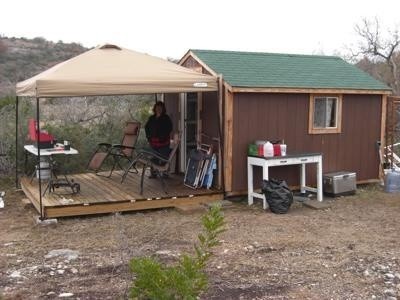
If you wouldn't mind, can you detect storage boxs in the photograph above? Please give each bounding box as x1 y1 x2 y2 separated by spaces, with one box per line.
323 171 357 198
29 119 57 148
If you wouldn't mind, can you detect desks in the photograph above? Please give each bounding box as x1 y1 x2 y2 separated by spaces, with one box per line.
24 143 79 197
247 152 322 209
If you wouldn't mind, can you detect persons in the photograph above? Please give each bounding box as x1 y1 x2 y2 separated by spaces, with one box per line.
143 99 172 179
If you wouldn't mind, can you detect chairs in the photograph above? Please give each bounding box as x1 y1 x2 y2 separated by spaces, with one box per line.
121 129 180 195
184 141 217 190
85 121 140 178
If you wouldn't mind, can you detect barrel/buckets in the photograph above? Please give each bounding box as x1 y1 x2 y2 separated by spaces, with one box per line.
384 167 399 192
36 162 50 179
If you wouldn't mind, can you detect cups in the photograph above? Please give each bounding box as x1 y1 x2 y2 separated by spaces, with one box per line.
64 140 70 150
280 145 286 156
